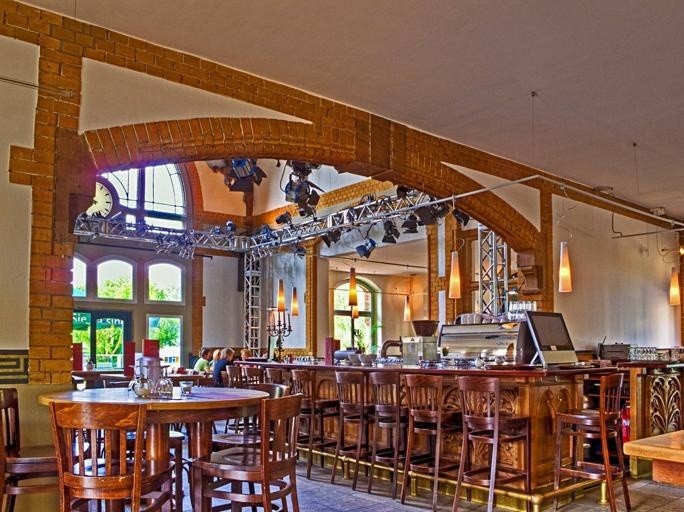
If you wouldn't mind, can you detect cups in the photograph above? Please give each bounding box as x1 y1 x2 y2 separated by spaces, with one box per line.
147 376 173 395
179 380 194 395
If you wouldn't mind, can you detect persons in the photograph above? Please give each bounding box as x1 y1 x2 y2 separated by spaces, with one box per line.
240 349 251 361
213 348 235 384
209 349 222 372
194 348 211 374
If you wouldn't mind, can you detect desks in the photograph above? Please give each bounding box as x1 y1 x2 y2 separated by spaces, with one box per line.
578 359 684 480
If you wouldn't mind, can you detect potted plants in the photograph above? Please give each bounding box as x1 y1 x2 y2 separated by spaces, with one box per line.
353 323 383 365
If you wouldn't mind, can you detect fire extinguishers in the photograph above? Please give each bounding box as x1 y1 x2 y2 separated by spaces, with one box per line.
621 403 630 446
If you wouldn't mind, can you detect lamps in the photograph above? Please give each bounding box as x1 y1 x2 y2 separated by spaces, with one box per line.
668 267 681 308
402 294 411 322
447 251 462 299
351 306 359 319
289 287 300 317
276 278 286 312
557 242 573 294
347 267 358 307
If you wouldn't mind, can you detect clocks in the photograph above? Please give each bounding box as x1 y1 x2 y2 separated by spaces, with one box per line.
83 176 121 220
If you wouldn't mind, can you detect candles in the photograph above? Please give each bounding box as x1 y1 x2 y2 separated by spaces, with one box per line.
266 309 292 329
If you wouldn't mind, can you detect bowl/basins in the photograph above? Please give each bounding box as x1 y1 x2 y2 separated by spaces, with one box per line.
347 354 361 365
358 354 377 366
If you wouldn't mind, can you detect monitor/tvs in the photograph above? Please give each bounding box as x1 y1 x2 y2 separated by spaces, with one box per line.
525 310 579 366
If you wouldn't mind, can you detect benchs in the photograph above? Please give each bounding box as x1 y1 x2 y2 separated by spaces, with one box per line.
621 429 684 490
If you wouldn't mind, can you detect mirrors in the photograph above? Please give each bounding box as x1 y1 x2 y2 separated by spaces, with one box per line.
253 240 306 361
